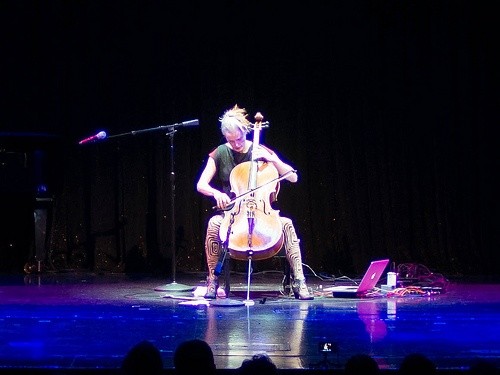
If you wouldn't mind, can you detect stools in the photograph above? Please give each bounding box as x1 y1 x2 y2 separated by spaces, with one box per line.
225 251 290 292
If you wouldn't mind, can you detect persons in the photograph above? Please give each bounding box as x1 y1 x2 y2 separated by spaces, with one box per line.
343 352 380 375
234 353 279 375
196 104 315 302
398 352 436 374
467 359 493 375
120 339 163 375
172 338 219 375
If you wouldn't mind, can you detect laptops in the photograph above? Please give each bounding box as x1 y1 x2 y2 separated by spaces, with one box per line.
323 260 389 294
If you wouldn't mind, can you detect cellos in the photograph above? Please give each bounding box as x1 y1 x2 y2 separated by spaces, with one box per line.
219 112 285 305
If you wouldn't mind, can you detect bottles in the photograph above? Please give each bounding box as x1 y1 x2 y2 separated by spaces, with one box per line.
387 272 397 285
387 301 396 314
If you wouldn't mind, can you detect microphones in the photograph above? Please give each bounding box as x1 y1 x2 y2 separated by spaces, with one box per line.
78 131 106 144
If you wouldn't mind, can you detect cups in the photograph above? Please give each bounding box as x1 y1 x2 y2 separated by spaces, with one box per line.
381 285 392 293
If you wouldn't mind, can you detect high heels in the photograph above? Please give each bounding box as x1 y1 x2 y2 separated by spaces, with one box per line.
204 275 219 299
292 276 314 300
